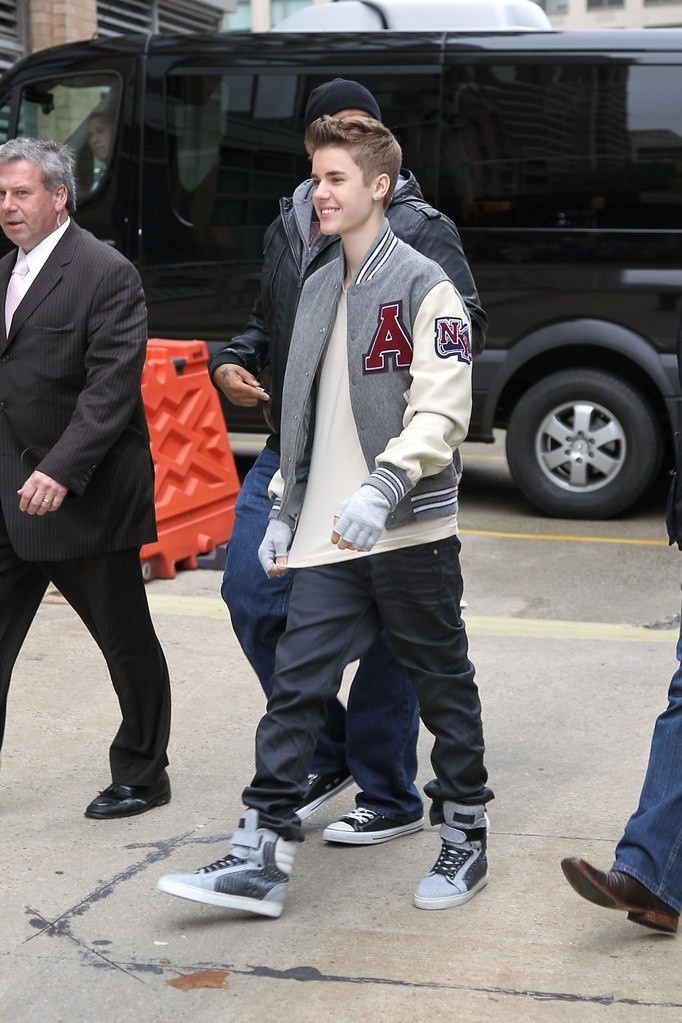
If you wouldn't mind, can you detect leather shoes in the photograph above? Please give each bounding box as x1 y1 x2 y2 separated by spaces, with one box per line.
563 857 679 931
84 783 171 820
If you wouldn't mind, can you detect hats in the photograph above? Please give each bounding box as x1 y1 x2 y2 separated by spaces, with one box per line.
304 78 382 132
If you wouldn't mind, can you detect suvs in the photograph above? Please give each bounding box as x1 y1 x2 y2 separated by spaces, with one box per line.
2 2 681 518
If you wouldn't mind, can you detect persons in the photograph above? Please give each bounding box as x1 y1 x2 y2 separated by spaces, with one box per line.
158 115 496 919
85 112 115 167
206 78 489 844
561 323 682 933
1 136 172 818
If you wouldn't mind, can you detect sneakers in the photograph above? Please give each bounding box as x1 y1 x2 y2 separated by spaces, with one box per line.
156 808 297 916
414 799 490 910
322 807 425 844
295 773 351 820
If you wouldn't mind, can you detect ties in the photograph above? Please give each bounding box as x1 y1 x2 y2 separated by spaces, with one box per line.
5 253 29 338
